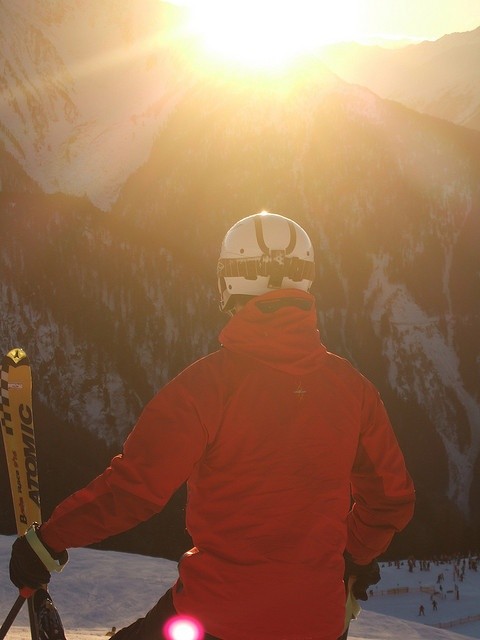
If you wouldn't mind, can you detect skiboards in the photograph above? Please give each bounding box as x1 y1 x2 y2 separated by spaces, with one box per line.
0 347 66 640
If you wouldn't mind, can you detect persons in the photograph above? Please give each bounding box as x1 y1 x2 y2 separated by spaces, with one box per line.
380 551 480 618
9 213 416 640
106 627 117 637
370 590 374 596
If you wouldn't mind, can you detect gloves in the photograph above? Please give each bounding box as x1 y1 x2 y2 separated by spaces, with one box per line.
345 558 381 601
9 527 69 589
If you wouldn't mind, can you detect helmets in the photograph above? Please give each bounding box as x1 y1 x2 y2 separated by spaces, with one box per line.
216 214 316 313
